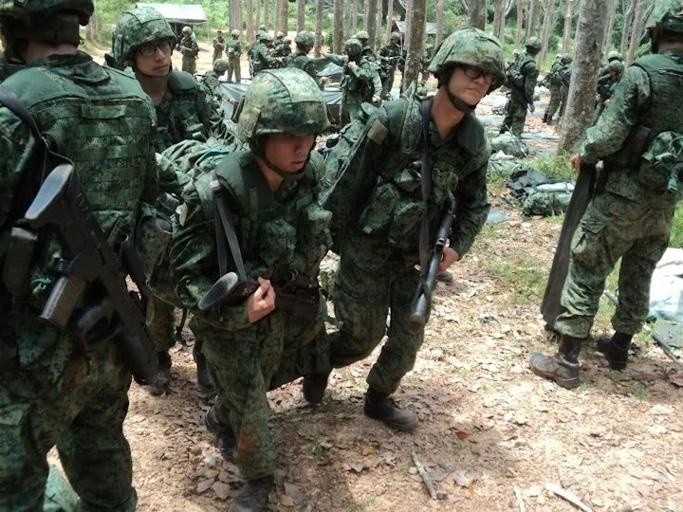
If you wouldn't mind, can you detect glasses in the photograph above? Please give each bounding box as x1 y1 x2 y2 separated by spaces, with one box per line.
137 40 172 57
456 64 498 87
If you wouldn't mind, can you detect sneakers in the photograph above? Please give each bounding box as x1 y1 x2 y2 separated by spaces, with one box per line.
436 269 453 283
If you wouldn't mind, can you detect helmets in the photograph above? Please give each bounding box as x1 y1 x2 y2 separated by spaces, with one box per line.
238 67 333 143
524 37 543 53
112 7 175 68
343 30 400 57
214 59 229 72
0 0 94 31
254 25 314 54
232 29 241 37
427 27 507 95
182 26 192 36
643 1 682 38
560 53 572 65
607 50 624 74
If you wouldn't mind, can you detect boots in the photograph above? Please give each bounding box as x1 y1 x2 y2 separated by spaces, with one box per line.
530 334 583 390
228 473 273 512
597 331 633 372
204 405 236 449
147 352 172 396
363 387 418 433
301 371 329 403
194 341 220 391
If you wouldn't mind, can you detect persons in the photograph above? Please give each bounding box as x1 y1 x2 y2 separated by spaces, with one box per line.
592 52 625 126
2 2 158 510
177 25 434 130
499 38 572 139
527 0 682 391
109 6 248 398
169 66 328 511
302 26 508 433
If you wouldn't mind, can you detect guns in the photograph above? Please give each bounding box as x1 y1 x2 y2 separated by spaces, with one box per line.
24 164 179 397
199 272 344 328
505 72 529 110
410 196 457 323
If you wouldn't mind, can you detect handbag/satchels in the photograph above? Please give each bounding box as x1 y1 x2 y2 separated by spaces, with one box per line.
524 183 572 217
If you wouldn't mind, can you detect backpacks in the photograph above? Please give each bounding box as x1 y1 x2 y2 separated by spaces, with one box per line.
319 92 491 213
136 139 326 312
